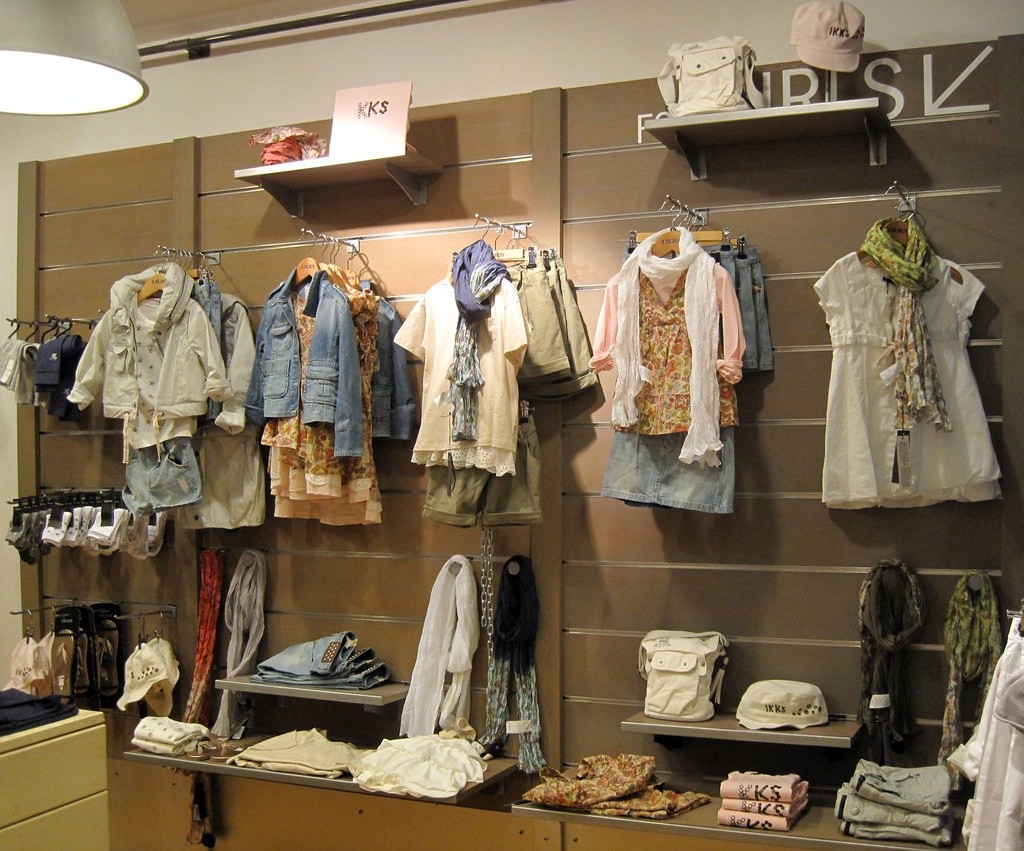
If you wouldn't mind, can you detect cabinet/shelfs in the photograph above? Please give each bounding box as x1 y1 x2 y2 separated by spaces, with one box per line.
122 673 519 804
511 708 958 851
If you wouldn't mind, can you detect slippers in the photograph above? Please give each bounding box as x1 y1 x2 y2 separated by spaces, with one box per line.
186 737 252 762
50 620 119 704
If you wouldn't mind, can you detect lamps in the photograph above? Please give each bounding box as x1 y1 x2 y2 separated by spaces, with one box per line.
0 0 149 117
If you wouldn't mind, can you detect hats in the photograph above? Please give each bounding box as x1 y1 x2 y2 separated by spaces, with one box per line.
0 630 55 693
116 637 181 718
735 678 830 731
789 0 865 74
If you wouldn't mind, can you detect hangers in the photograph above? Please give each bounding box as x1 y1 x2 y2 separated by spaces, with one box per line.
137 247 216 303
290 230 381 302
858 185 963 285
449 217 557 271
618 199 757 259
8 316 97 358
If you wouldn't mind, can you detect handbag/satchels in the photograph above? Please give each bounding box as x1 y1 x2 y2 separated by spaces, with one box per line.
656 35 768 119
637 629 730 723
